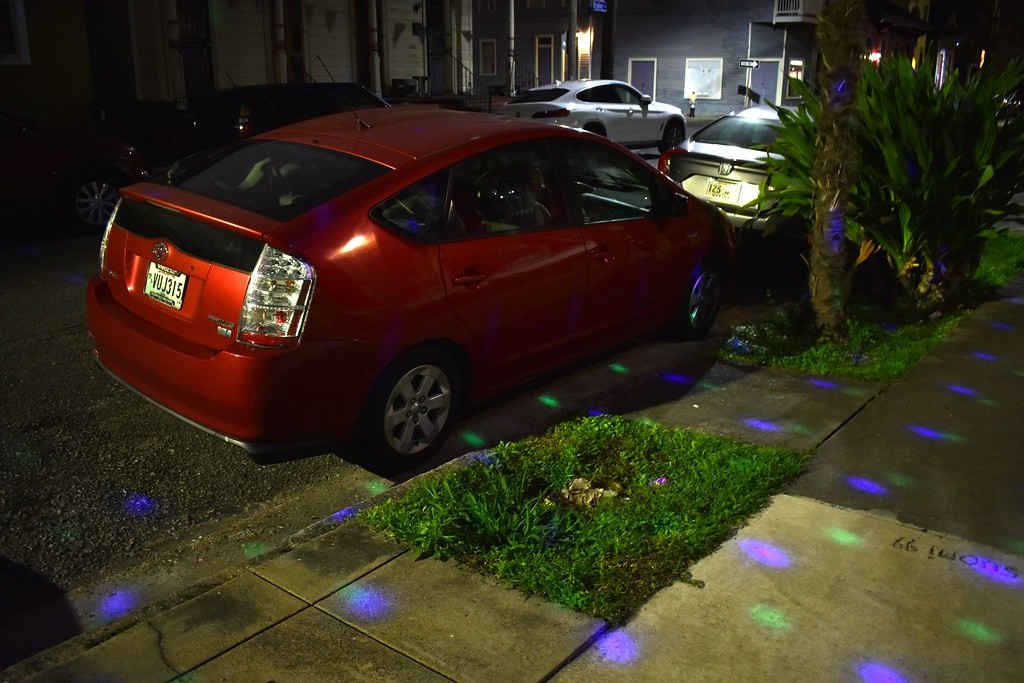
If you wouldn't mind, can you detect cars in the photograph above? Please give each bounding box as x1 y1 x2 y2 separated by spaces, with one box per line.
653 104 827 236
82 105 736 470
0 109 148 231
151 83 388 156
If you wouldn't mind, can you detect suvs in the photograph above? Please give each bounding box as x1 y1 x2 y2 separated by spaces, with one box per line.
502 78 687 158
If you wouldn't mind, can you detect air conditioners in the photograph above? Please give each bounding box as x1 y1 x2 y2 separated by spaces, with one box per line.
772 0 824 25
